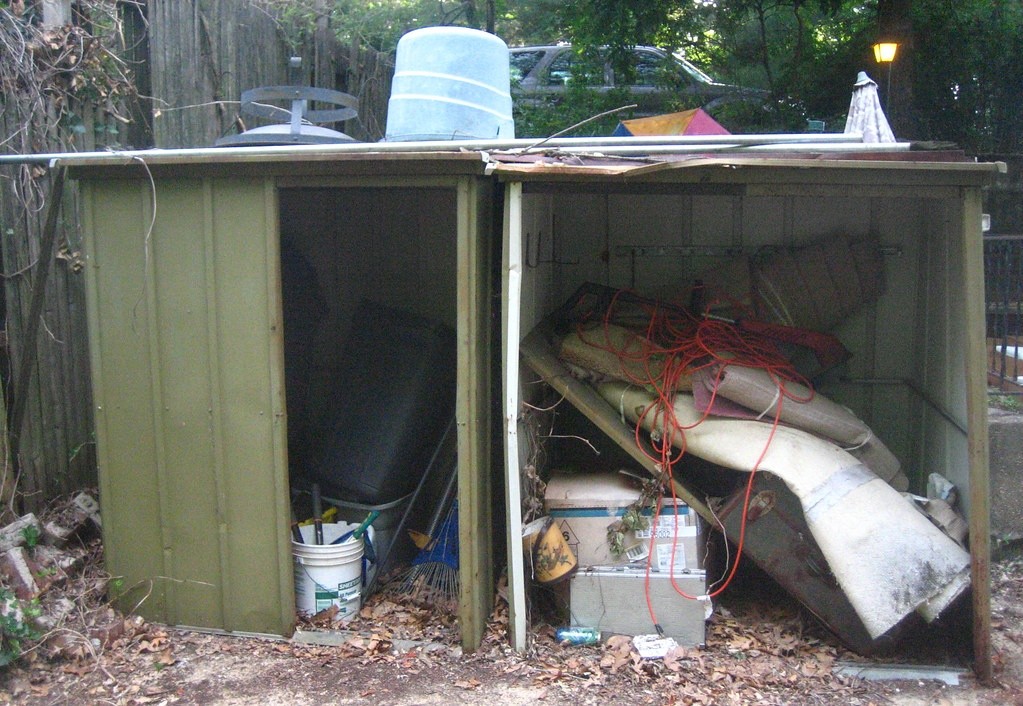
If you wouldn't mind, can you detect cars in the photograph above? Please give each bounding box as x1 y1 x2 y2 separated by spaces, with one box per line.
510 42 809 135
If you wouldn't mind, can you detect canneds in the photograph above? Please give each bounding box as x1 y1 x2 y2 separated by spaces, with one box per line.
556 627 601 646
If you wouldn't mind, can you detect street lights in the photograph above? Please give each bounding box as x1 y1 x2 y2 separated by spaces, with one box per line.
869 28 902 129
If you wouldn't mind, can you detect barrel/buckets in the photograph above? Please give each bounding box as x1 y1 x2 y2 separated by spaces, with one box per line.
385 24 514 150
291 523 365 623
